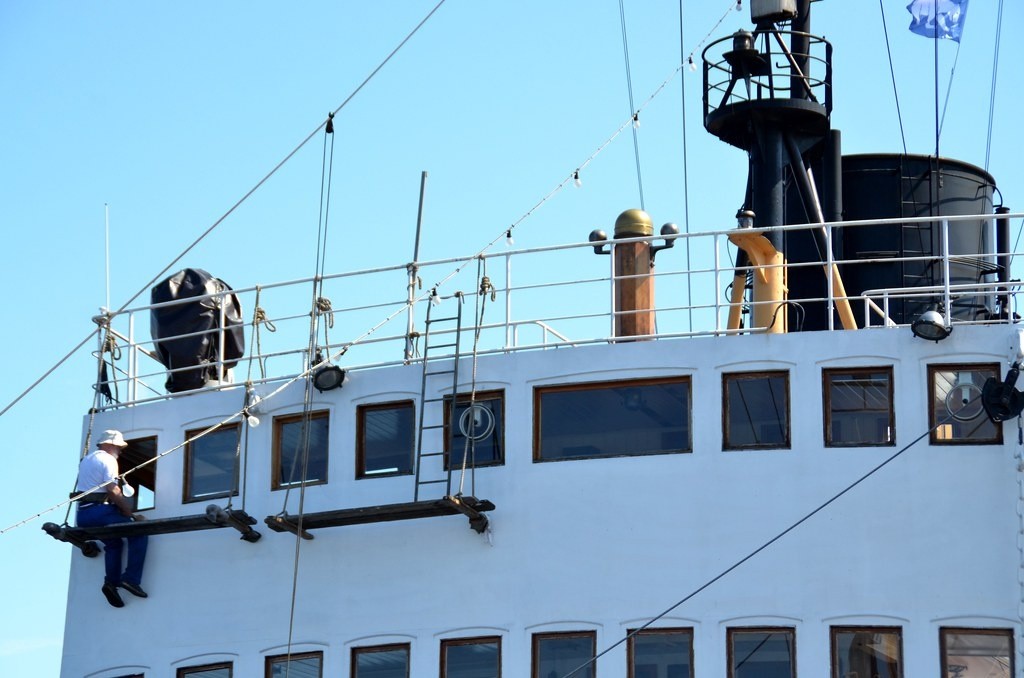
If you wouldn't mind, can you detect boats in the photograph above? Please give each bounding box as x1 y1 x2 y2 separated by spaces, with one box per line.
41 0 1023 678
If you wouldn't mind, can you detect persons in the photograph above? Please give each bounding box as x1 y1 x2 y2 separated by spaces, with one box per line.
73 429 148 608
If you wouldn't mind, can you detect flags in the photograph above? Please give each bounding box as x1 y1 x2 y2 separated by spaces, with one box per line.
906 0 969 43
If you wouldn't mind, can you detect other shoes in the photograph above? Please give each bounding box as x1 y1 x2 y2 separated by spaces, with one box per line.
102 583 124 608
118 576 148 598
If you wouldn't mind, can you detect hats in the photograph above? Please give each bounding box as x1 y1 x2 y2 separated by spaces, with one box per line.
96 430 128 449
